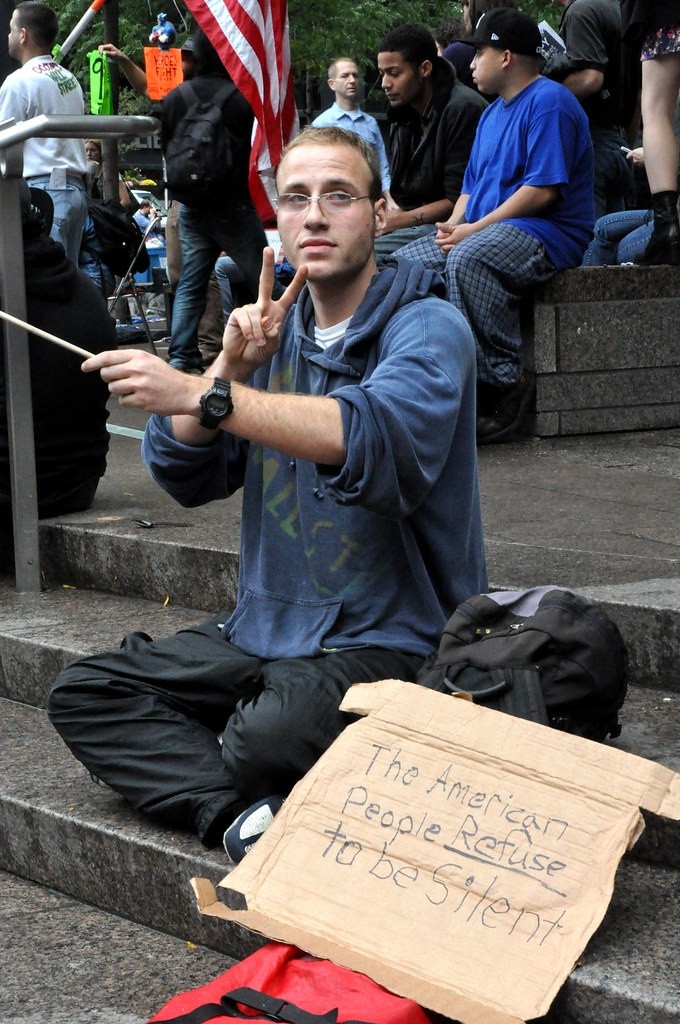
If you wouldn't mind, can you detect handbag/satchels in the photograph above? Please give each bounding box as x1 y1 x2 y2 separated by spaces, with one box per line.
86 200 150 277
143 943 433 1024
418 585 629 741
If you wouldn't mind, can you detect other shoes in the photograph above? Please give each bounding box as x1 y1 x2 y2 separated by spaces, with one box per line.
114 319 148 338
131 310 166 322
167 355 206 376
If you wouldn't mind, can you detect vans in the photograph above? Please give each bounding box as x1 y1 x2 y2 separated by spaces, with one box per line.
129 189 170 229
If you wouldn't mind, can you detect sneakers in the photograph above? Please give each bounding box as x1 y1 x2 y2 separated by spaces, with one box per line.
476 374 532 442
220 794 288 866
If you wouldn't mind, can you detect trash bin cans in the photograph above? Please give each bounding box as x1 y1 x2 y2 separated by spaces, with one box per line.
132 248 167 283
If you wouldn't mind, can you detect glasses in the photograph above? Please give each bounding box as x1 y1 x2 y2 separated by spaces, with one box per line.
270 191 374 215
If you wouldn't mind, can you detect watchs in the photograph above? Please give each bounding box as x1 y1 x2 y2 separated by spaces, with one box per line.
200 376 234 429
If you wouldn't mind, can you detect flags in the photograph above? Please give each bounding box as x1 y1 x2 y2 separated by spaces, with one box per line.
183 0 302 220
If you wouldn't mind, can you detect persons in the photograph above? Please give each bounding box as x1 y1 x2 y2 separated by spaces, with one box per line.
85 135 130 298
0 1 88 269
540 0 680 268
434 0 514 103
132 198 166 246
312 55 392 207
373 24 490 266
0 185 122 521
388 8 596 445
43 122 486 865
98 23 284 374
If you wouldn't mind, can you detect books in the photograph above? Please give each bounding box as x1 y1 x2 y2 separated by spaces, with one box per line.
537 20 567 63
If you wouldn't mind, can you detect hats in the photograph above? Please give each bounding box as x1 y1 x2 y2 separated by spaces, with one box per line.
452 6 544 61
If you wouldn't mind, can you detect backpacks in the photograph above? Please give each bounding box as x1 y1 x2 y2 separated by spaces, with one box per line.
162 80 236 206
1 185 116 517
